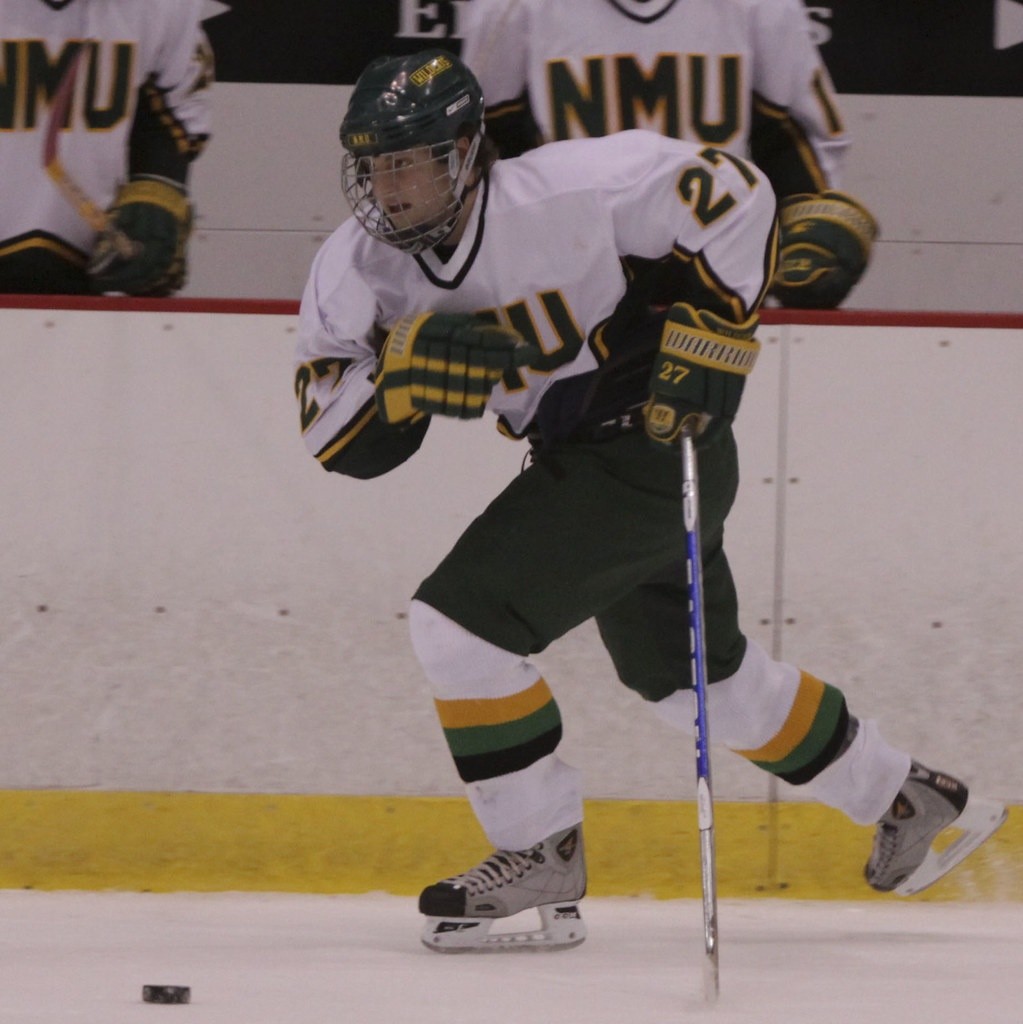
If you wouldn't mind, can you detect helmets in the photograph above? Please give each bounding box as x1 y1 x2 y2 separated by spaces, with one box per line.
339 48 486 255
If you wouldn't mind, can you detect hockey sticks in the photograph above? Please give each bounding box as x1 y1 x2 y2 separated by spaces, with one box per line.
43 39 113 228
683 432 719 1006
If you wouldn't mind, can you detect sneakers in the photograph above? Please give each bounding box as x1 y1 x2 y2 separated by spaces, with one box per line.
864 761 1004 897
419 822 587 953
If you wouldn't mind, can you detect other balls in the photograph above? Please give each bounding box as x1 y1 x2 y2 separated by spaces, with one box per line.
142 985 191 1005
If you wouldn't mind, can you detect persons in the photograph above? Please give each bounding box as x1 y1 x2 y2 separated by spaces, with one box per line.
293 48 1009 954
0 0 214 297
397 0 880 311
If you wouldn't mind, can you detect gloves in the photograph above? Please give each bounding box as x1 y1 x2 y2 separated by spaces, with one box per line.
86 174 194 297
766 190 878 309
643 302 760 441
375 312 519 431
